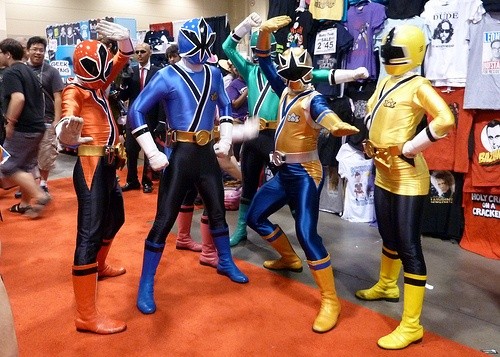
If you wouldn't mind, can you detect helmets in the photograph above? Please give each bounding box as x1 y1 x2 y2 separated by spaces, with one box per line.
380 25 426 76
178 17 216 64
74 40 113 88
277 47 313 91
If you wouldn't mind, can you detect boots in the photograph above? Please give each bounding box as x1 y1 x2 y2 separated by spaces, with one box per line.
378 273 429 350
355 246 402 302
97 238 126 281
137 238 165 314
199 213 222 268
261 224 303 272
230 196 252 247
210 225 248 284
178 203 203 251
306 252 341 333
71 262 127 334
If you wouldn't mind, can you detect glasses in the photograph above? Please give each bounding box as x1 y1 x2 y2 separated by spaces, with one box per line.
135 50 150 54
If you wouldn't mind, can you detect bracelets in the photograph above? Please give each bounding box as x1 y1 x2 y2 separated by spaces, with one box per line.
3 113 18 123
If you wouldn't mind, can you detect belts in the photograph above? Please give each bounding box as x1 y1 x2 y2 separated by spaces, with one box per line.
268 150 319 166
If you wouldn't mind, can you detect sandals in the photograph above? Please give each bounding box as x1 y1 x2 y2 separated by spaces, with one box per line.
10 202 31 214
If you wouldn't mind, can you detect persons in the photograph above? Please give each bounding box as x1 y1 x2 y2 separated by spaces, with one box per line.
0 37 65 220
55 20 134 336
166 44 181 64
356 23 454 349
118 42 248 206
0 275 19 357
223 13 368 247
128 16 248 314
177 54 219 269
243 16 361 334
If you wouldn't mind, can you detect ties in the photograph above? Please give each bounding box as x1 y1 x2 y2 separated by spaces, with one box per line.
139 67 145 90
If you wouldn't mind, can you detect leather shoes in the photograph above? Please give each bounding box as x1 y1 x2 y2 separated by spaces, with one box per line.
143 183 153 193
120 183 140 191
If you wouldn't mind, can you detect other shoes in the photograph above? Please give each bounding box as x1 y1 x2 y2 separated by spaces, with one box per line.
39 184 48 193
14 191 23 197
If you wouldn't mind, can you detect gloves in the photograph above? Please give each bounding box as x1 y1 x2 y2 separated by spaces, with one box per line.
214 117 235 158
57 117 94 146
95 20 134 56
326 66 368 86
403 123 448 159
230 12 262 43
315 110 361 137
132 125 169 171
254 15 291 58
